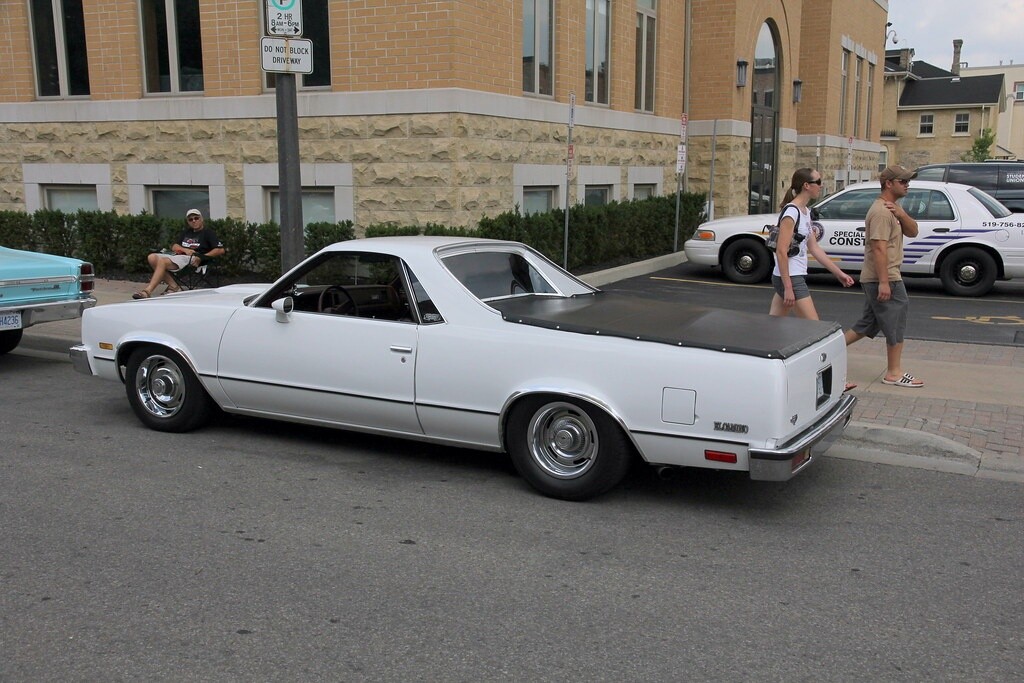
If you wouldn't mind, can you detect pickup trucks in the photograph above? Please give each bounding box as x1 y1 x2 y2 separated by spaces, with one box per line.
69 236 857 501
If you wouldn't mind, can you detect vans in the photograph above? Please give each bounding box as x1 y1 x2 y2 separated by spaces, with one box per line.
899 159 1024 218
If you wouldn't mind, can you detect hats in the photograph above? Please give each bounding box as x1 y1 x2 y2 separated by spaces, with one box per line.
880 164 918 184
186 209 202 217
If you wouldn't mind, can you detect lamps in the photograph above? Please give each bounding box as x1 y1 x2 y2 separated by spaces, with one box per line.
884 22 898 48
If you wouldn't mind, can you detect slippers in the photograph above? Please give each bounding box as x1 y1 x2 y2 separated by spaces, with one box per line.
882 373 925 387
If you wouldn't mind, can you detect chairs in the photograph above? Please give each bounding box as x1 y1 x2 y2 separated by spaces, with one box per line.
158 245 220 294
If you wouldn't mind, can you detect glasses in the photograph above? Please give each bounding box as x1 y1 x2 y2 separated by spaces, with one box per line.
807 178 821 186
188 217 200 221
892 180 910 185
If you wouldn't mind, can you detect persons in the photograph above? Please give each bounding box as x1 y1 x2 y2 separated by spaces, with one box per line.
769 167 857 392
844 164 923 387
132 209 224 300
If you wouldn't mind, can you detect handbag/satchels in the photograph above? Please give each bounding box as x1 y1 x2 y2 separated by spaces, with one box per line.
762 204 806 257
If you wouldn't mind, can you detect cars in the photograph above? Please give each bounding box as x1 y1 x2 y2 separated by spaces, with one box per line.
0 243 97 353
684 180 1024 297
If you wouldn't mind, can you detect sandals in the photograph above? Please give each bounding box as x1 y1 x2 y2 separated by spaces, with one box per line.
132 289 152 299
162 285 182 295
845 382 856 391
